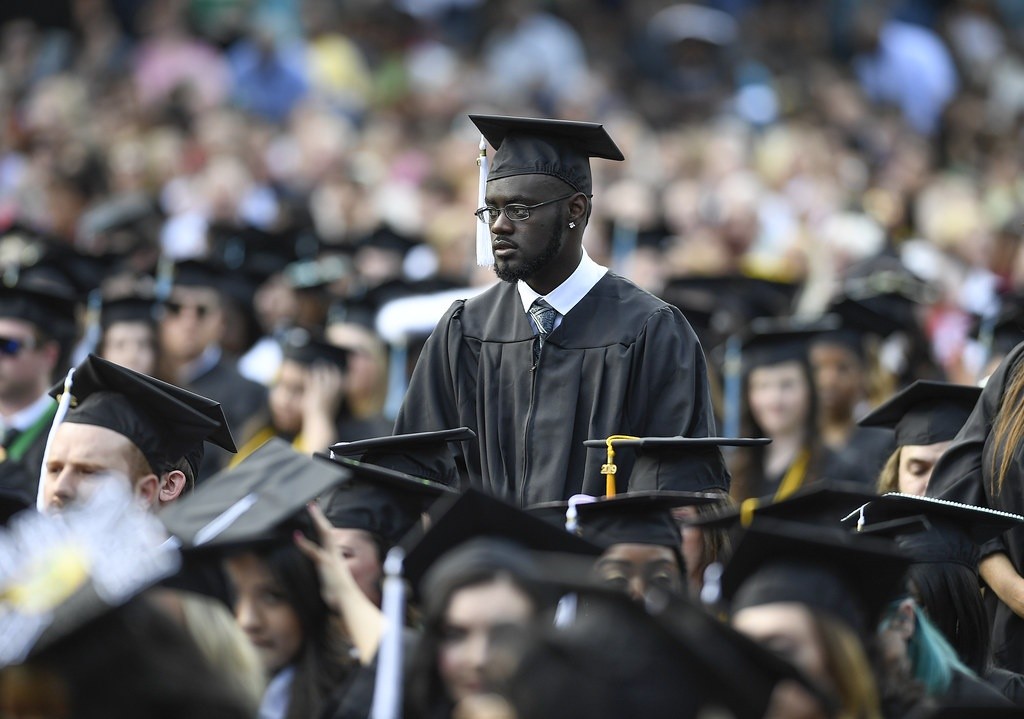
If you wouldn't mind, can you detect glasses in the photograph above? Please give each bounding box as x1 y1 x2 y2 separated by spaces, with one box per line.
474 191 593 225
0 335 41 359
165 300 222 319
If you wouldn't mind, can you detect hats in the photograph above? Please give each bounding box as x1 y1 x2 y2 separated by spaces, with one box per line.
270 316 355 377
654 269 923 360
2 194 168 329
26 351 1020 716
467 110 628 195
206 204 472 329
148 255 258 298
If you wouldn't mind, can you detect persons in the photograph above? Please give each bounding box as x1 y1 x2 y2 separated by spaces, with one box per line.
0 0 1023 719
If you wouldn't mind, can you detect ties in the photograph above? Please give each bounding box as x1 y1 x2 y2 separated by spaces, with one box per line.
529 303 559 375
